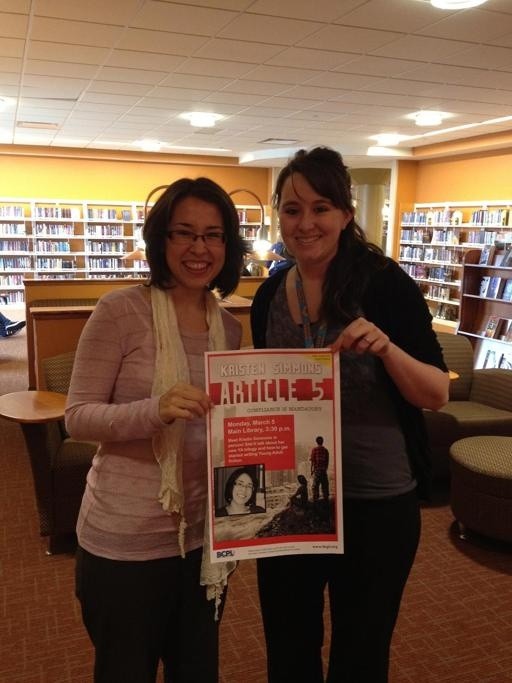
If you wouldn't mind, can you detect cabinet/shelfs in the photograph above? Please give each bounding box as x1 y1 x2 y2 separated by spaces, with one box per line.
0 200 35 302
456 249 512 340
134 203 151 277
398 222 512 326
85 203 133 279
236 204 274 276
34 200 86 281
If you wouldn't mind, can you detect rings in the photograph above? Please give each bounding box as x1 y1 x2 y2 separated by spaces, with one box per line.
363 336 370 344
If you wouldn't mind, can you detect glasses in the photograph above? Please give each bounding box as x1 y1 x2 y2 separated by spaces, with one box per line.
166 228 228 247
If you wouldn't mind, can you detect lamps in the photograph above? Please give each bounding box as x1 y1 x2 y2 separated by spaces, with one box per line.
137 183 272 256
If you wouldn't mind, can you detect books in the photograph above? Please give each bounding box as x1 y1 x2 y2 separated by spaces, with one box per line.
0 205 254 304
400 208 511 371
203 347 346 566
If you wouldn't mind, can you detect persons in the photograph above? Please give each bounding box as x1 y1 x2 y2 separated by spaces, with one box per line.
242 250 261 276
250 147 449 682
265 238 289 277
309 436 330 501
216 468 265 517
0 311 26 337
289 475 307 506
64 174 244 683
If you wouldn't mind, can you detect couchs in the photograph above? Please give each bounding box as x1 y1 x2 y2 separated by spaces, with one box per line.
450 435 512 540
22 349 101 558
416 332 512 490
